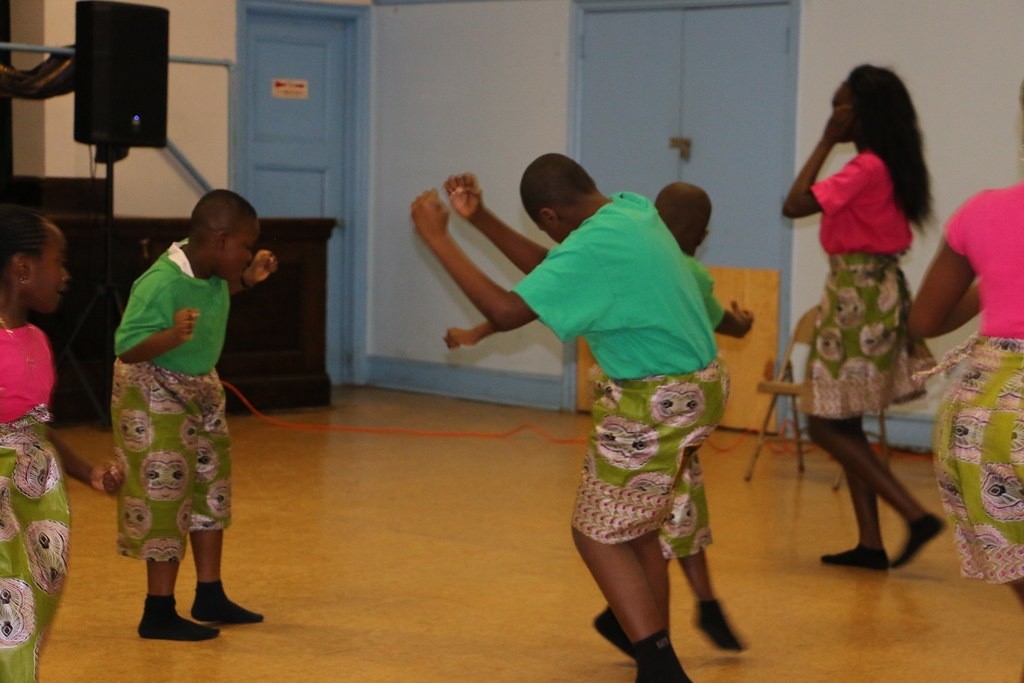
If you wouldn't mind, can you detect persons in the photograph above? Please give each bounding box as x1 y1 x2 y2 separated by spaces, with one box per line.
781 63 946 570
442 183 754 661
410 156 733 683
907 179 1024 604
111 190 277 641
0 205 128 683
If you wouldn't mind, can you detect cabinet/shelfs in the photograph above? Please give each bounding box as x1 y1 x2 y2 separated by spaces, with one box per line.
0 176 337 426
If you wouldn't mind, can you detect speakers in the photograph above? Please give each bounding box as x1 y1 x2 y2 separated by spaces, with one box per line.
73 0 169 148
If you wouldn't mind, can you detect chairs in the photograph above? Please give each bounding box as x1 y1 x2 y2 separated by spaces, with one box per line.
744 305 886 491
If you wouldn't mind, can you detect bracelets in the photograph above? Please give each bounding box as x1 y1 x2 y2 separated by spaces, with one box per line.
240 277 250 289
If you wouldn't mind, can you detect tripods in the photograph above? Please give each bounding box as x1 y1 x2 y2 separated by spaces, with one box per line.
52 146 122 429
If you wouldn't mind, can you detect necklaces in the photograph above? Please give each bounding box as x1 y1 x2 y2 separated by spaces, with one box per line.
0 317 34 364
